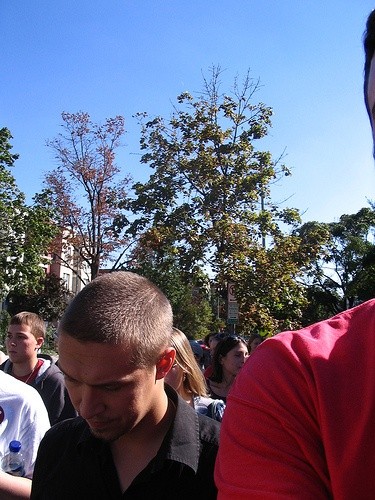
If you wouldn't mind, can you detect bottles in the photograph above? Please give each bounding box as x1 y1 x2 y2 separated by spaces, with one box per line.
4 440 26 477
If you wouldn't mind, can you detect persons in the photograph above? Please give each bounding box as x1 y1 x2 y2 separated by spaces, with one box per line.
0 311 78 427
0 350 8 365
188 329 265 403
0 370 52 500
164 325 226 424
212 8 375 500
30 266 223 500
